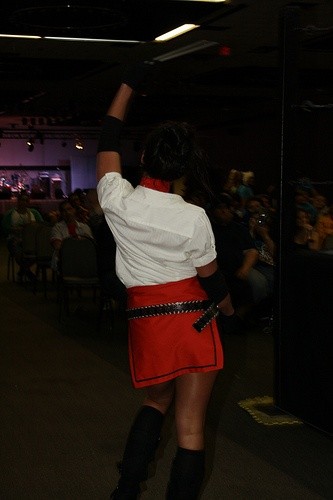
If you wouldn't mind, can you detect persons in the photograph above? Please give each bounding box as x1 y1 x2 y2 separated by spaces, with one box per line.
0 121 333 341
94 70 245 500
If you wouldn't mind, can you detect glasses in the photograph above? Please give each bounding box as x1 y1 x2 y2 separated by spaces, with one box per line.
216 206 230 209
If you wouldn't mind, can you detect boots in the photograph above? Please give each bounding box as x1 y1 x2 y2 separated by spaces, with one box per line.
166 448 207 500
109 405 164 500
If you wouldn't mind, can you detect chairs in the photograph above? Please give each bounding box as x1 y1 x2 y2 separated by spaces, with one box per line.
7 235 105 323
